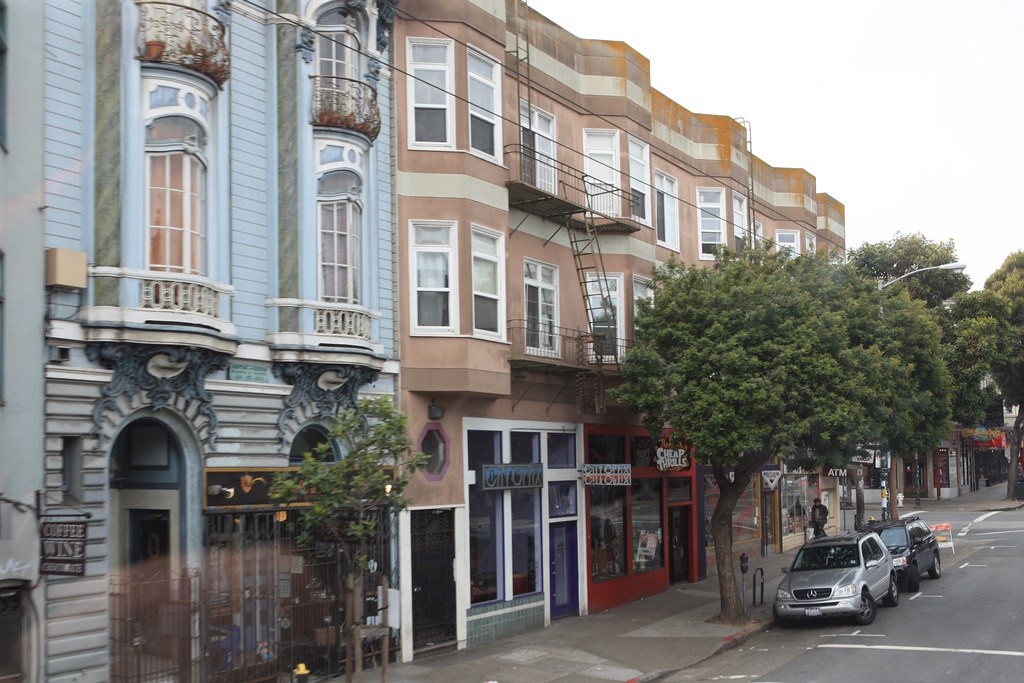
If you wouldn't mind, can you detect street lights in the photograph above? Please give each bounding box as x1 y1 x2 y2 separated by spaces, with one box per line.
878 261 967 520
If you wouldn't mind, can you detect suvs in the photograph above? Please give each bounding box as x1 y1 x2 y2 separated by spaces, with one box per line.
859 517 941 593
773 530 899 625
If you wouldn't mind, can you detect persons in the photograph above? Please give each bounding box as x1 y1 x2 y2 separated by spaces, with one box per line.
812 498 828 538
790 498 805 517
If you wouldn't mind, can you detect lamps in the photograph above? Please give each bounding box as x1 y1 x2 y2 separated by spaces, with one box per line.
427 398 446 419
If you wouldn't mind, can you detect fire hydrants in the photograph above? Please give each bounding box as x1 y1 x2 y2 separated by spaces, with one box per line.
896 493 905 508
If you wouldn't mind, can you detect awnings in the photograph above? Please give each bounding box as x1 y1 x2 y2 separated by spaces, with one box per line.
824 461 868 478
976 429 1007 450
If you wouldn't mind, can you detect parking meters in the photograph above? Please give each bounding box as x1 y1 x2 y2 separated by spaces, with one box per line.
741 553 748 613
